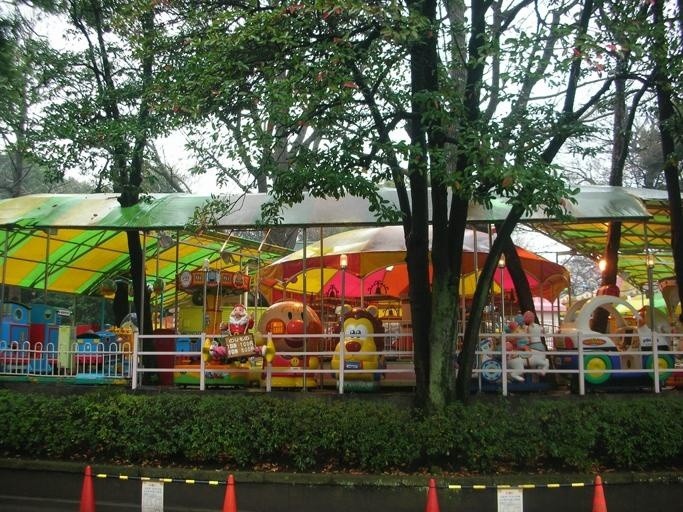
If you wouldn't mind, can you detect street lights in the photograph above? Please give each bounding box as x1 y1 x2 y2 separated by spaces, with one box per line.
202 258 210 334
647 254 655 331
340 255 346 333
498 253 506 333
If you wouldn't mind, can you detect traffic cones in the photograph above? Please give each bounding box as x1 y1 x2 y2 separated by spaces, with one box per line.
80 466 96 512
423 480 441 512
593 475 607 512
223 475 237 512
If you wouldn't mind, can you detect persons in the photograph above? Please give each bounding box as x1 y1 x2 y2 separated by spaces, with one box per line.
221 304 255 337
